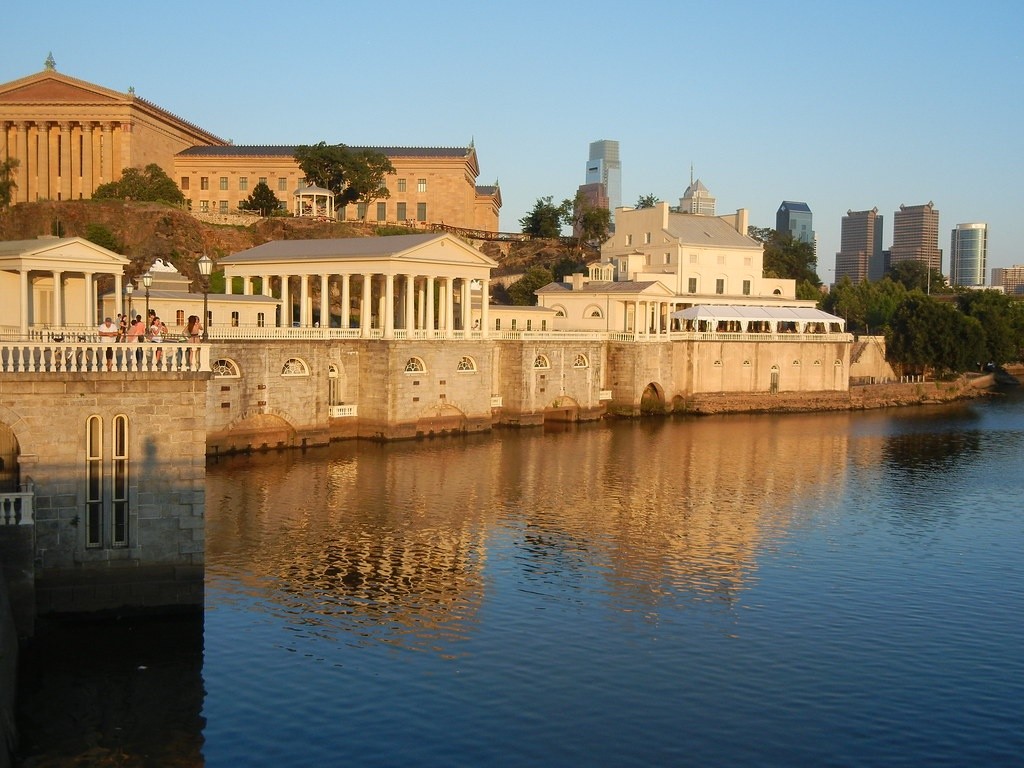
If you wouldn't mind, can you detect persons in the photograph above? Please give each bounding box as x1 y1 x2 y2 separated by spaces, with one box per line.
182 315 203 370
127 319 139 343
118 315 127 343
147 309 156 337
116 313 122 328
194 316 201 325
150 318 168 365
136 314 145 342
472 319 480 330
98 317 118 372
687 320 827 334
314 320 320 328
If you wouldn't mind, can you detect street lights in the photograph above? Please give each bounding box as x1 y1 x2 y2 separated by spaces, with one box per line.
125 277 134 331
140 269 153 334
196 255 214 343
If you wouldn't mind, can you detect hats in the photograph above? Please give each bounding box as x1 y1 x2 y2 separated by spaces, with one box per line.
106 318 111 322
